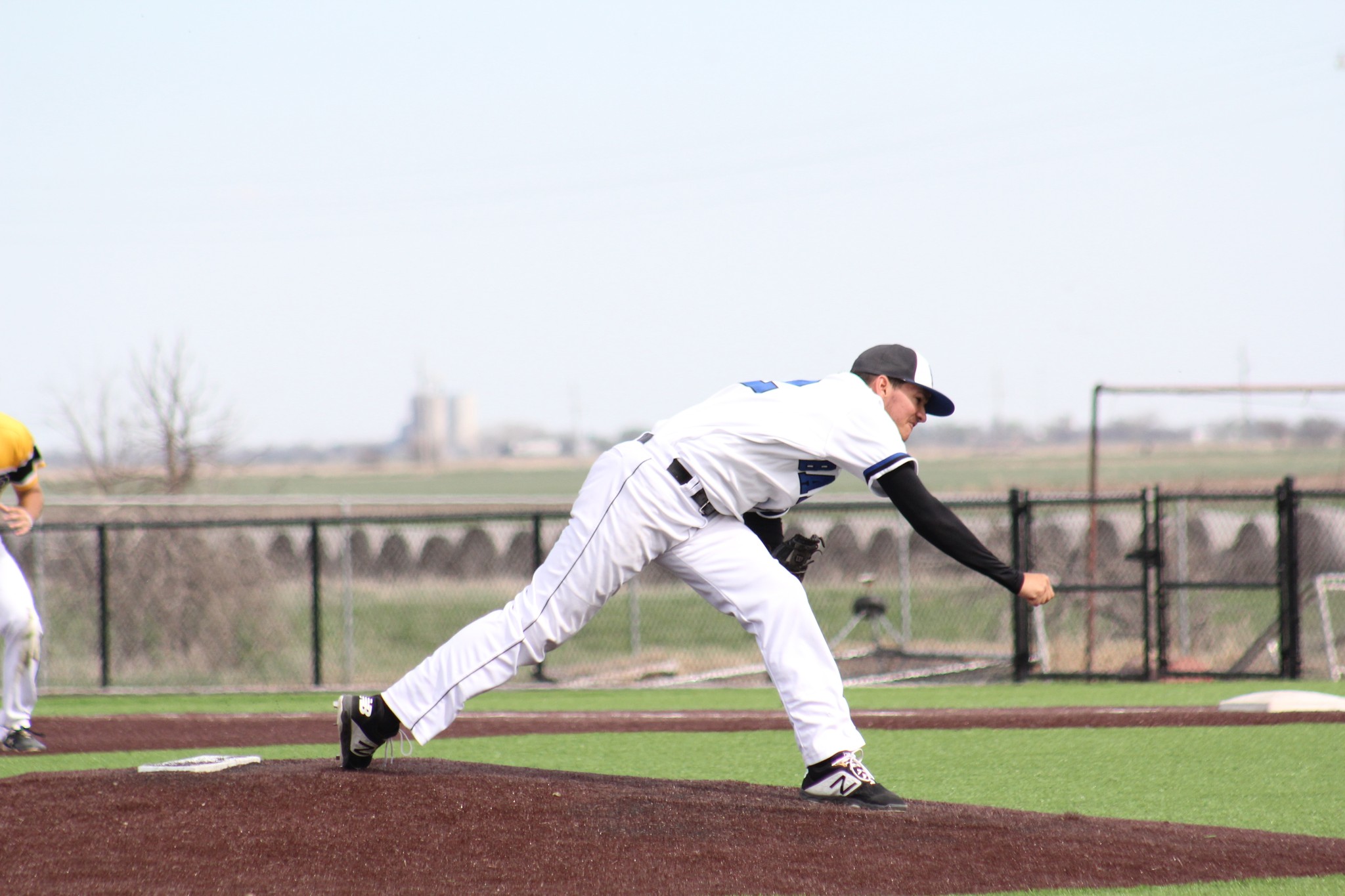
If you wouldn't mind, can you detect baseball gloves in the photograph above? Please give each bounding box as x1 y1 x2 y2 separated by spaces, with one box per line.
769 531 826 583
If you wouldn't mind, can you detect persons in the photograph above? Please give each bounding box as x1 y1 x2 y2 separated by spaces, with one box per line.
0 417 52 755
331 339 1054 811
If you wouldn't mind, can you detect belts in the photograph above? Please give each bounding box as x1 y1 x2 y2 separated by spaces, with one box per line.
638 433 716 517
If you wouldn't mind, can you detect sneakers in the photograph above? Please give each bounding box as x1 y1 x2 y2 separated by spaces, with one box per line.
333 694 412 771
799 748 908 813
2 726 47 753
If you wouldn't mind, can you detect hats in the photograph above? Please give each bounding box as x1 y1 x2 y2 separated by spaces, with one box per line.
850 344 955 417
